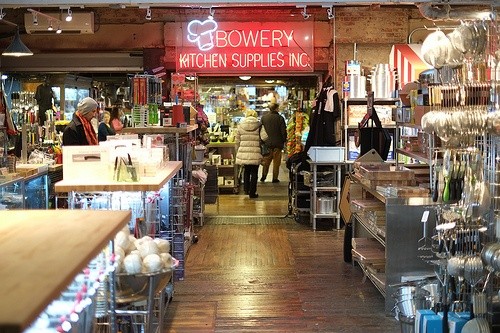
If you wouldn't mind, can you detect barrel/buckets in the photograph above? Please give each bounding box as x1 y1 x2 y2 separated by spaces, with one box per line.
193 149 205 162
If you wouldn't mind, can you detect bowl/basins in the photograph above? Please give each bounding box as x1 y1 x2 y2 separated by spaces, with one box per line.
227 137 233 142
113 256 180 298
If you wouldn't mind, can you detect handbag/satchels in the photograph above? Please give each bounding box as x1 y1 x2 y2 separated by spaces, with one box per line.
258 123 272 155
111 118 124 131
358 107 391 161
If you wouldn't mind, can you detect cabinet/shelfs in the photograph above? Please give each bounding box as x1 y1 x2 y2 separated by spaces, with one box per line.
0 85 500 333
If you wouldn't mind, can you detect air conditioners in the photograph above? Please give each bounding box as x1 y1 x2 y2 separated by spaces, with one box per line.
24 13 94 35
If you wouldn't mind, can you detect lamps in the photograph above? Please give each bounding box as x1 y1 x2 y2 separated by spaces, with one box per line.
65 10 72 21
208 10 216 19
265 80 274 83
48 19 54 30
33 15 38 25
327 7 334 19
146 9 151 20
56 25 61 33
186 77 196 80
301 7 309 19
239 76 251 81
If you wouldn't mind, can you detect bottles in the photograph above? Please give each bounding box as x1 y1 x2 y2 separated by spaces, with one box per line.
7 154 16 173
312 191 336 214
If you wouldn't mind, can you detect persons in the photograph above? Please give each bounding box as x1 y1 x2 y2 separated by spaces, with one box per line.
261 103 287 183
62 96 99 146
110 107 124 133
33 80 59 126
234 110 269 199
98 111 116 141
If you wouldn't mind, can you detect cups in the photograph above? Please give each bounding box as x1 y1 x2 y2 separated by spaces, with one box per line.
219 138 226 142
112 162 141 182
212 139 217 142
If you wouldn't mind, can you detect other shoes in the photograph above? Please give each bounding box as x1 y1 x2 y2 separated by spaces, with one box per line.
245 191 249 195
250 194 257 197
272 179 279 182
261 176 266 181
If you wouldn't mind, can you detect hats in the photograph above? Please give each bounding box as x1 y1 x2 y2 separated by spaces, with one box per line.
77 97 98 116
245 110 257 118
270 103 279 111
